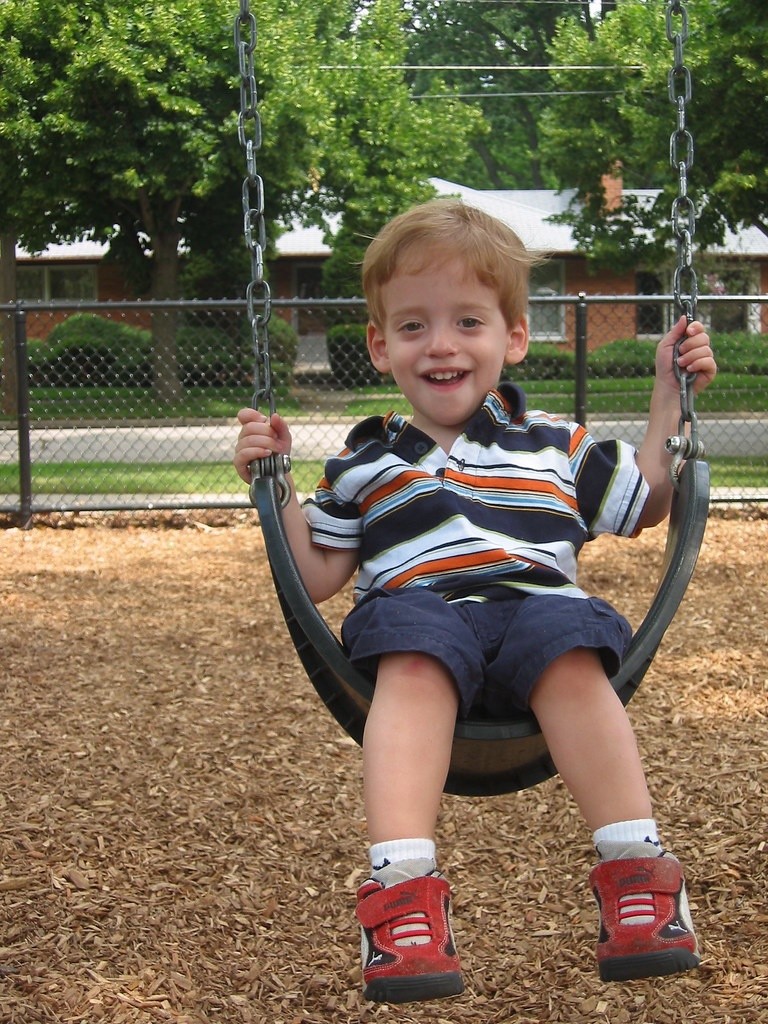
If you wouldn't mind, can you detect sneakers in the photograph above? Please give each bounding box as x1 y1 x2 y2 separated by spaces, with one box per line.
357 858 464 1005
590 840 701 982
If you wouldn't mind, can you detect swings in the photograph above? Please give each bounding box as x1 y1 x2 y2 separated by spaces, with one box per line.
232 1 713 800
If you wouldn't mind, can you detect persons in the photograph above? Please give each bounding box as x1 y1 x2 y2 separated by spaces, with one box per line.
234 200 717 1003
